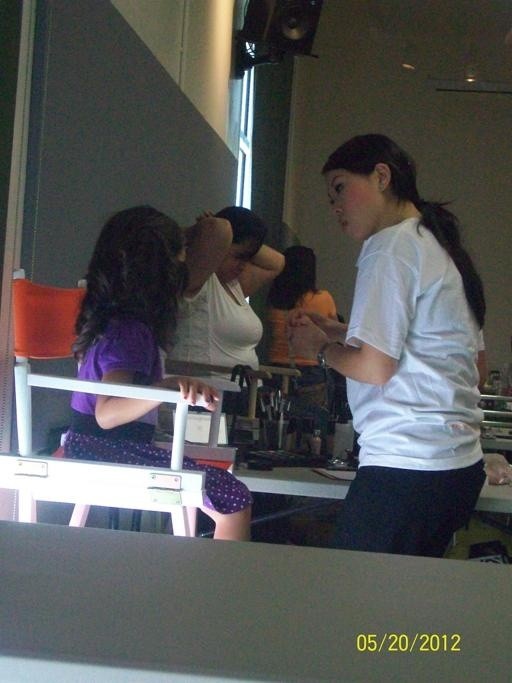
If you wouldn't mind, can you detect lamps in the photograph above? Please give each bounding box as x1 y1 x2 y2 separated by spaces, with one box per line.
454 64 476 82
399 44 421 69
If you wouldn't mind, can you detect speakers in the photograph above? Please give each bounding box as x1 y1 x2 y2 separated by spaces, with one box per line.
241 0 324 57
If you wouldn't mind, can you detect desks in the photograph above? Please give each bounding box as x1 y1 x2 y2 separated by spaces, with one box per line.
480 426 512 451
233 463 511 529
0 521 511 681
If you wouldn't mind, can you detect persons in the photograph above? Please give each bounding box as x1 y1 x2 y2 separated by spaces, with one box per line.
285 134 489 558
266 245 339 402
61 204 254 542
158 206 286 415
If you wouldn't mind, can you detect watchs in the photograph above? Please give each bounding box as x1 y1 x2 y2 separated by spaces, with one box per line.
317 342 344 369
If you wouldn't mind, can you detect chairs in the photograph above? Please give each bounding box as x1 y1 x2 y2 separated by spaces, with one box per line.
156 342 302 463
12 269 243 537
